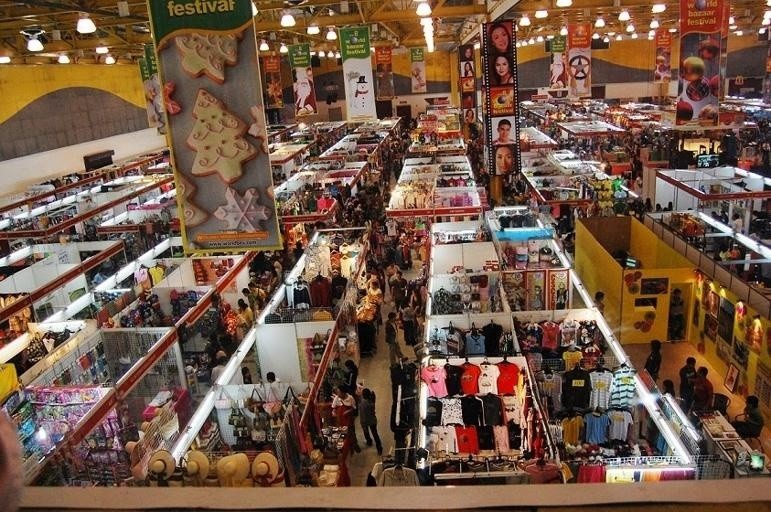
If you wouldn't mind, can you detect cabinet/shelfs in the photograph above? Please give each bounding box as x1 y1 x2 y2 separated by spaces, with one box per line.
0 96 771 488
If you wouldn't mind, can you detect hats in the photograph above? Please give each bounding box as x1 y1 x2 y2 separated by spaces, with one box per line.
183 451 278 484
125 408 176 479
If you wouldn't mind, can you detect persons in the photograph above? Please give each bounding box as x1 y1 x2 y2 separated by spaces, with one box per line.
646 198 653 212
733 395 765 439
736 214 744 233
465 109 475 123
690 365 713 425
729 214 738 231
751 230 762 242
242 366 253 383
679 354 697 414
595 291 606 315
331 384 362 455
217 236 307 358
492 119 517 145
646 339 662 380
91 269 109 287
462 47 473 61
496 145 517 175
502 175 531 205
360 388 385 456
383 117 417 184
463 62 474 76
267 370 280 383
669 288 687 342
344 359 359 416
712 211 720 220
370 259 429 345
489 24 513 53
210 355 227 382
466 123 490 197
492 54 515 85
721 210 729 224
656 203 663 211
662 378 675 398
340 180 392 258
386 312 408 369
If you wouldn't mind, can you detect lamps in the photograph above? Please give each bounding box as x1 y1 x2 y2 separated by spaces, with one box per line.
96 43 108 54
25 35 45 52
76 10 96 34
106 54 116 65
416 2 435 54
251 0 375 59
513 0 667 47
57 52 70 64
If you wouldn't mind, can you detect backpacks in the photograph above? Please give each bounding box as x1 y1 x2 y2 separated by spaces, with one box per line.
120 295 170 328
170 289 217 383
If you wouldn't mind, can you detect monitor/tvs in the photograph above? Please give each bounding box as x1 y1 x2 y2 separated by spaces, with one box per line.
696 154 720 169
626 258 638 268
749 454 765 471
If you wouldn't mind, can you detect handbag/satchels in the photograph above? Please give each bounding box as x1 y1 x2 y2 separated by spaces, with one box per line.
229 386 311 442
310 329 332 368
